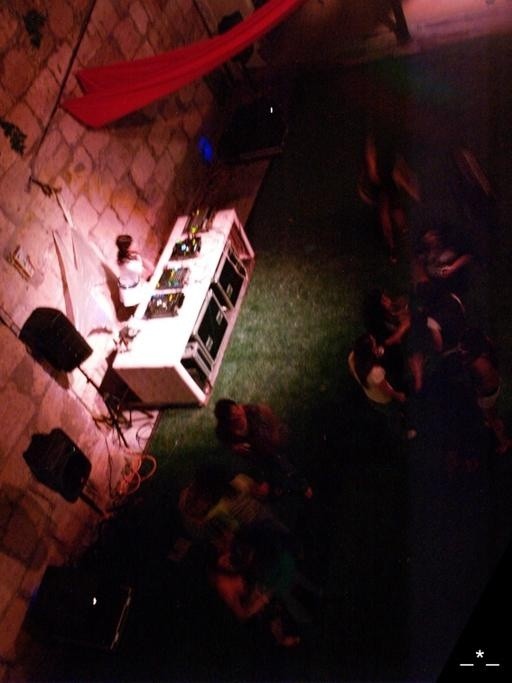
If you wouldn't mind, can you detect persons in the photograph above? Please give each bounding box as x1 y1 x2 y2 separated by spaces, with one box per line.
83 234 155 316
346 116 508 460
178 397 316 646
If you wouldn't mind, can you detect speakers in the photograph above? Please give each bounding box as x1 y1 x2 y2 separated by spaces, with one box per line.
23 428 92 503
18 307 94 373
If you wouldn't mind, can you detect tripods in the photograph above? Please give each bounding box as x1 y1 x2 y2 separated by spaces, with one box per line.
78 366 154 448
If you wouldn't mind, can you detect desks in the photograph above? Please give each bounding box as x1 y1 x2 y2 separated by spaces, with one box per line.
111 207 257 408
